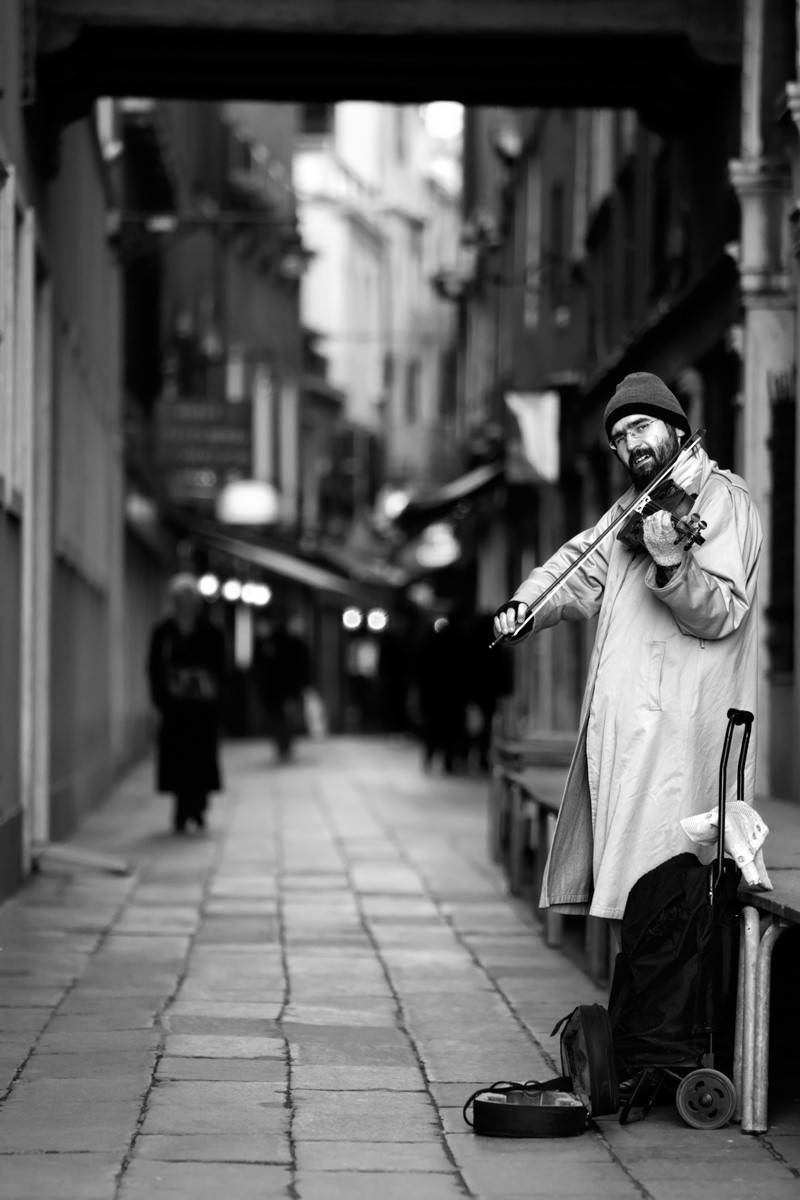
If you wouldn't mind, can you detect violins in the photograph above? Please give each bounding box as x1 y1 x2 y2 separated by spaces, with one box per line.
616 478 708 552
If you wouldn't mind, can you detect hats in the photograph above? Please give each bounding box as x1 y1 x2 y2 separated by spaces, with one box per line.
603 372 691 444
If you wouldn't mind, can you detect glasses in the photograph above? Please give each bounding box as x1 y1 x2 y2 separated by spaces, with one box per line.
608 418 658 449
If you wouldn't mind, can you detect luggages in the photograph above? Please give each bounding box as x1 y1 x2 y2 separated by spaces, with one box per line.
602 708 755 1125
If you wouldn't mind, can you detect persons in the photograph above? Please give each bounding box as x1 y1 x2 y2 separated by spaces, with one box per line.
495 374 766 1111
147 575 518 828
